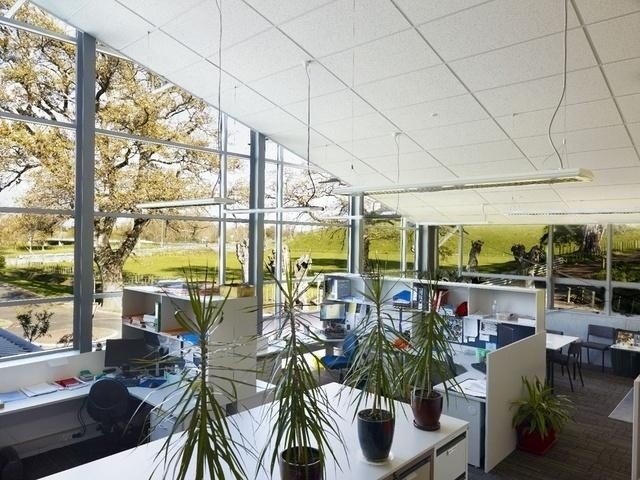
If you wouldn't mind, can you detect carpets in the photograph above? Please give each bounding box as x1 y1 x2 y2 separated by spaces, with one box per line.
608 387 633 424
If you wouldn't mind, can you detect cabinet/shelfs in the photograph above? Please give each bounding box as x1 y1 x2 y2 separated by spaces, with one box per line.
323 272 545 328
435 392 485 469
149 409 183 441
121 285 258 409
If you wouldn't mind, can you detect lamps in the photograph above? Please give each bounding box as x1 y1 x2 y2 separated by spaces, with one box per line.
327 2 593 195
136 2 236 209
317 130 404 221
221 58 324 213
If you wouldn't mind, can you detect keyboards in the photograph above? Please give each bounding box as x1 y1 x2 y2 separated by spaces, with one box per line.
117 378 141 388
471 362 486 374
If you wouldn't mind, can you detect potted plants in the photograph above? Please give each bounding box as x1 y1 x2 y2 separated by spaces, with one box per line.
258 265 352 479
394 266 470 431
504 371 579 453
337 265 414 462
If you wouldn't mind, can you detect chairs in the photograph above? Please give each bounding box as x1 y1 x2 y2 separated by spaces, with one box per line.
546 329 584 387
86 377 145 451
579 323 615 373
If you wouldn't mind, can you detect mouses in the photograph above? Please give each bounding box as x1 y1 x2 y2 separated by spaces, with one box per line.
147 380 159 389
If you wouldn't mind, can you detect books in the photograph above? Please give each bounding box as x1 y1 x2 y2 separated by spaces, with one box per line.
53 376 89 392
413 286 457 314
61 379 80 387
20 383 59 398
123 300 160 332
324 278 352 301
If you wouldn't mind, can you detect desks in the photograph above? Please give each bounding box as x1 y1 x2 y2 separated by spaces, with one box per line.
1 368 208 430
256 328 487 404
37 382 470 480
545 333 580 393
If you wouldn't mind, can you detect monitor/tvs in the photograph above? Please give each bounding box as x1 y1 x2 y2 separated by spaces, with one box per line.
321 302 346 327
495 323 515 348
104 338 146 378
